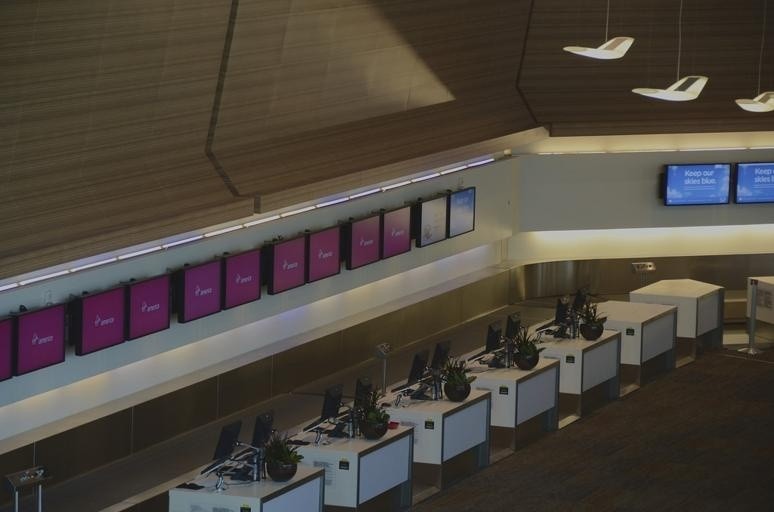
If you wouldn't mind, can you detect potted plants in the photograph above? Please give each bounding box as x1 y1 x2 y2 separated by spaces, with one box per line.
440 363 476 402
358 392 390 441
580 298 608 341
265 437 304 483
510 326 545 371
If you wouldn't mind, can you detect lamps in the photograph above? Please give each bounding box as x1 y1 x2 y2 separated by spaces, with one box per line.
562 1 634 60
633 1 710 102
735 0 773 114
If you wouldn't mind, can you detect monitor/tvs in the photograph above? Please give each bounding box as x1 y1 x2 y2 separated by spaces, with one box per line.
407 340 451 387
380 205 411 260
221 247 261 310
70 285 126 356
486 311 521 354
556 287 587 322
321 379 372 418
213 413 274 459
663 161 774 206
343 214 380 270
126 274 171 340
265 233 306 296
414 193 448 248
306 225 341 283
448 186 476 239
0 303 65 383
176 259 223 323
